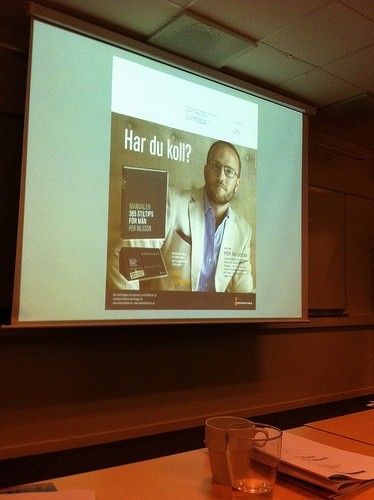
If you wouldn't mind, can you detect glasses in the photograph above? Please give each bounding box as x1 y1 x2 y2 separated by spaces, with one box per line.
206 158 240 180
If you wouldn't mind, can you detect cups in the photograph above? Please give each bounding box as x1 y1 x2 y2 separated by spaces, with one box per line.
204 416 269 486
224 422 283 494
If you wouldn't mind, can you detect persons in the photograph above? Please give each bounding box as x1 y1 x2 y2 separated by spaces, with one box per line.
109 140 255 295
